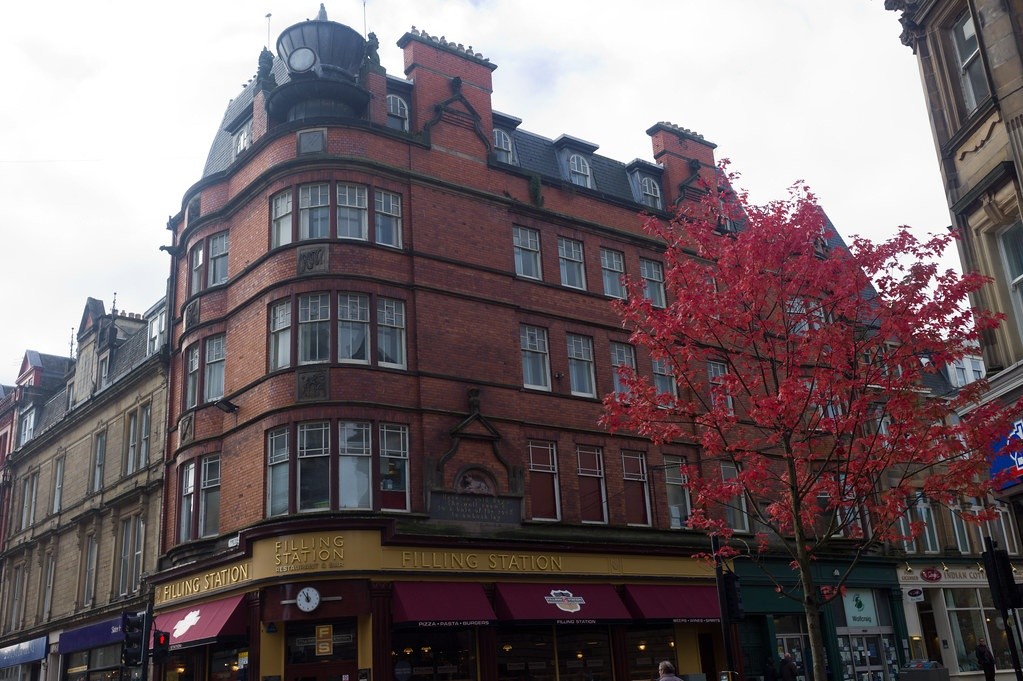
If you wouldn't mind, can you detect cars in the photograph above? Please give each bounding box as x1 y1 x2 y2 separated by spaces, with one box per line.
902 660 944 670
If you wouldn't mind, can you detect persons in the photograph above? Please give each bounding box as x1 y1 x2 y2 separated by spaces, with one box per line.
657 661 684 681
975 637 997 681
764 653 799 681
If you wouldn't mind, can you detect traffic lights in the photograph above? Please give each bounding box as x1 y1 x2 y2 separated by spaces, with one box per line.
123 614 145 665
153 629 170 664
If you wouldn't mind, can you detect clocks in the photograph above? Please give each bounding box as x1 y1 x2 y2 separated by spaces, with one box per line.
296 587 319 613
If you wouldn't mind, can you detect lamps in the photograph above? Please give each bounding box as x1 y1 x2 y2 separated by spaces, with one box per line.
942 561 948 571
833 569 839 577
404 648 413 655
576 650 583 659
976 563 983 571
213 397 239 413
667 637 674 647
636 641 647 650
503 644 512 652
906 562 912 572
1010 563 1017 572
421 647 431 652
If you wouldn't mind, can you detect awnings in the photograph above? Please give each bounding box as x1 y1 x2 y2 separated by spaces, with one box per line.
148 592 249 655
495 583 633 624
392 582 498 628
623 584 721 624
